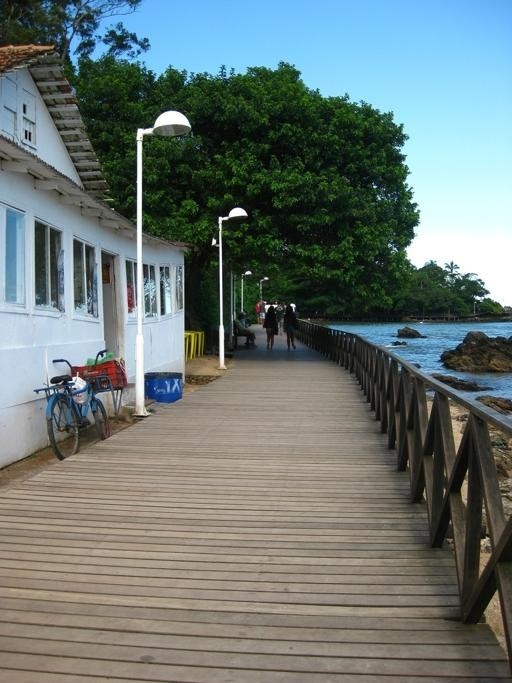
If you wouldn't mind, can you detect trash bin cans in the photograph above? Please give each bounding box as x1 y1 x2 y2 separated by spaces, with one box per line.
145 370 183 403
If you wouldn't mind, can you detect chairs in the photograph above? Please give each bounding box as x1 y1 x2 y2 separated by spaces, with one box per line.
233 320 251 349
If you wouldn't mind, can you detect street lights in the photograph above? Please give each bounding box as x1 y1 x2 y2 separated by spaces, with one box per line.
258 277 270 301
132 107 193 419
240 269 254 321
214 205 249 373
473 301 477 315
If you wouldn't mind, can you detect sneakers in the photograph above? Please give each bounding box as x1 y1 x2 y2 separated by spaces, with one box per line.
244 341 296 351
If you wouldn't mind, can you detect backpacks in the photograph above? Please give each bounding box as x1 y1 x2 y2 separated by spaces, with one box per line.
256 302 261 313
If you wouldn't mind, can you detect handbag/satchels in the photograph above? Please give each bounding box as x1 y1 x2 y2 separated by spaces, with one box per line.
263 313 267 328
274 322 278 335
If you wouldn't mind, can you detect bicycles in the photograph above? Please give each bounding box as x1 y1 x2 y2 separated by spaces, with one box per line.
32 346 118 462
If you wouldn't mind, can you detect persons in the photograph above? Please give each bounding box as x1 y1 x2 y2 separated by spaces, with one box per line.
282 305 298 351
233 310 257 348
259 298 298 335
262 305 277 349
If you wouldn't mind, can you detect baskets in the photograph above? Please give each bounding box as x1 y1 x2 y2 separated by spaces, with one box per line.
69 359 129 390
